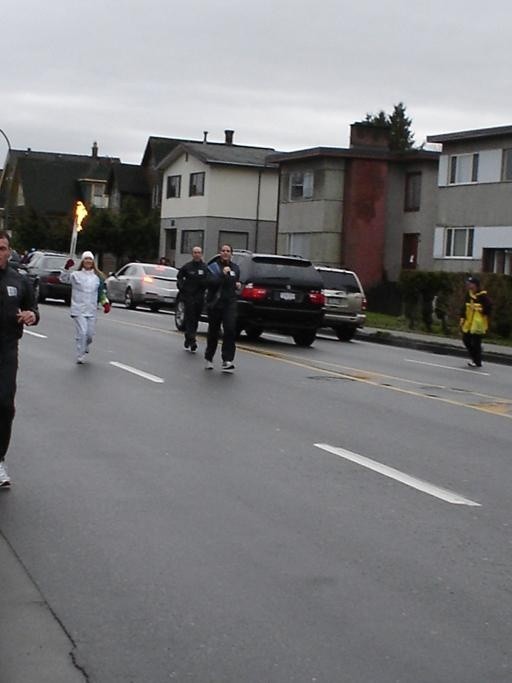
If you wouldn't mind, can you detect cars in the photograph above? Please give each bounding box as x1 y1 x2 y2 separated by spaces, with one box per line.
102 258 181 312
16 245 104 303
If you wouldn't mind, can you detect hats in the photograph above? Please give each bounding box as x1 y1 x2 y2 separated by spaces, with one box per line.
82 251 94 260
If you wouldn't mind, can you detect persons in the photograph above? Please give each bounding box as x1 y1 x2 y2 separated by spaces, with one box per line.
160 257 166 266
200 244 242 370
177 247 208 352
58 251 111 364
0 230 40 490
459 278 490 367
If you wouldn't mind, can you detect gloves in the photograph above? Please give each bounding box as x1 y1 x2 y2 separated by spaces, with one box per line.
65 259 74 268
103 303 110 313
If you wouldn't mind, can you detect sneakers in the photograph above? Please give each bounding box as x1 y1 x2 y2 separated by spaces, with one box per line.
468 361 477 366
206 360 213 369
222 361 235 369
0 460 12 486
184 340 198 353
77 355 86 364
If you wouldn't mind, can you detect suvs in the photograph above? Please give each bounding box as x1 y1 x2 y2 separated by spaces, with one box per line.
170 245 370 351
